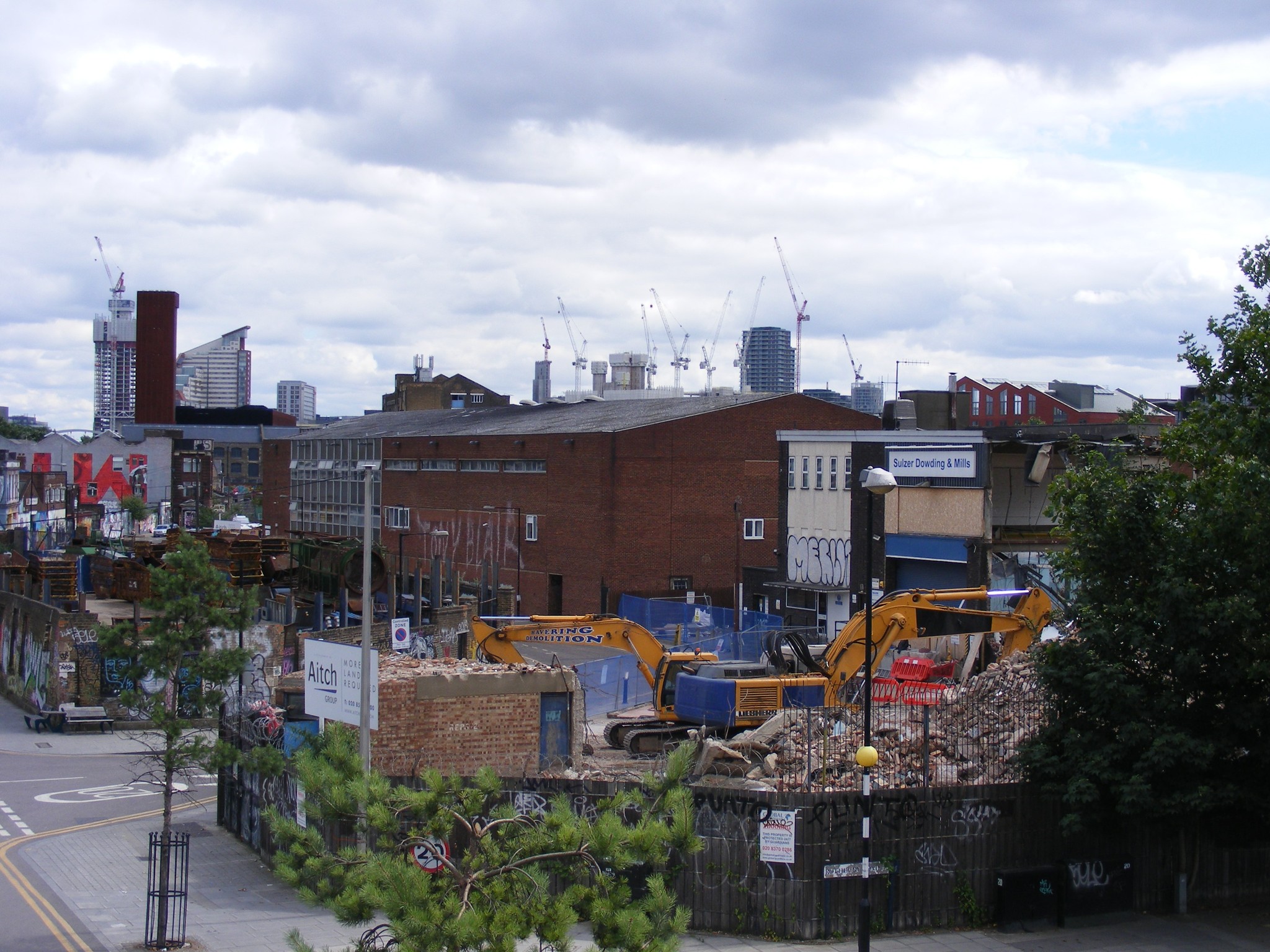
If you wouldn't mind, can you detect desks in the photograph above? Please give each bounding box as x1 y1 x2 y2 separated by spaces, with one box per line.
41 711 67 732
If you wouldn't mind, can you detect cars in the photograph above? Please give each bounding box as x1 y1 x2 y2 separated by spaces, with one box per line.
170 524 178 529
153 524 171 537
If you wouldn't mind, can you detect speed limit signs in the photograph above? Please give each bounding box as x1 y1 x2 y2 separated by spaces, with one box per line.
414 834 449 872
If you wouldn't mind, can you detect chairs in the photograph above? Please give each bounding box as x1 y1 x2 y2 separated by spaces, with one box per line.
23 704 53 732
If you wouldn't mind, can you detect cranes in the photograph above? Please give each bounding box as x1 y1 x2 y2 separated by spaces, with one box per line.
640 304 658 390
842 334 863 387
650 288 691 388
700 290 732 397
733 276 766 394
558 297 588 401
541 317 551 361
774 236 810 393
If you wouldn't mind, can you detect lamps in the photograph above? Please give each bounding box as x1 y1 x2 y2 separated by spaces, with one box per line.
914 481 930 488
392 442 401 446
429 441 439 446
470 441 479 446
872 534 884 541
514 440 525 445
963 541 977 548
563 440 575 446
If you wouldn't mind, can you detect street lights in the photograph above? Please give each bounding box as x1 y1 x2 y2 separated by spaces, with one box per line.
398 530 450 618
280 495 304 531
30 463 66 550
857 464 898 952
483 505 521 617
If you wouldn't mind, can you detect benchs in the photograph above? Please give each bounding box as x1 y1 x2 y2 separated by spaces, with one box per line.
61 706 114 735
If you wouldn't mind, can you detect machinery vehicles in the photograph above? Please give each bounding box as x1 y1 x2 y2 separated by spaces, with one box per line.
466 612 720 758
674 586 1054 742
